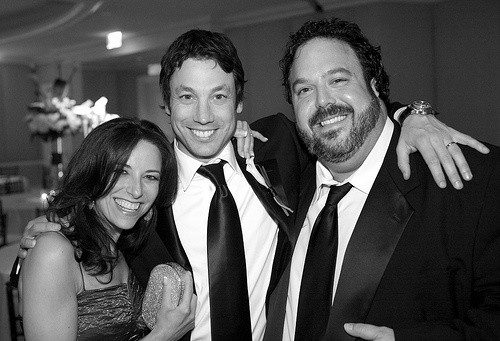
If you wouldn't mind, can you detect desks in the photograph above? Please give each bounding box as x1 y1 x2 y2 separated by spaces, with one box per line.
0 189 59 241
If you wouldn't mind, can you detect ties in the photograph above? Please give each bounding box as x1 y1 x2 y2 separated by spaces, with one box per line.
293 182 354 341
197 160 252 341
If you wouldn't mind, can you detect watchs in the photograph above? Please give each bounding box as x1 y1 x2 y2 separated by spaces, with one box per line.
398 100 435 125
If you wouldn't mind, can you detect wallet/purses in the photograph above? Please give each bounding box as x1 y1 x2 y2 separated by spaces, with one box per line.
142 263 187 331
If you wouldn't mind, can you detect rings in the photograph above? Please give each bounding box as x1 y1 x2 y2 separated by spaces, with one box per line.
446 141 456 147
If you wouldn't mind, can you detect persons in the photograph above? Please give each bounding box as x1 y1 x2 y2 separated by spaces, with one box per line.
264 17 500 341
15 116 270 341
23 27 490 341
